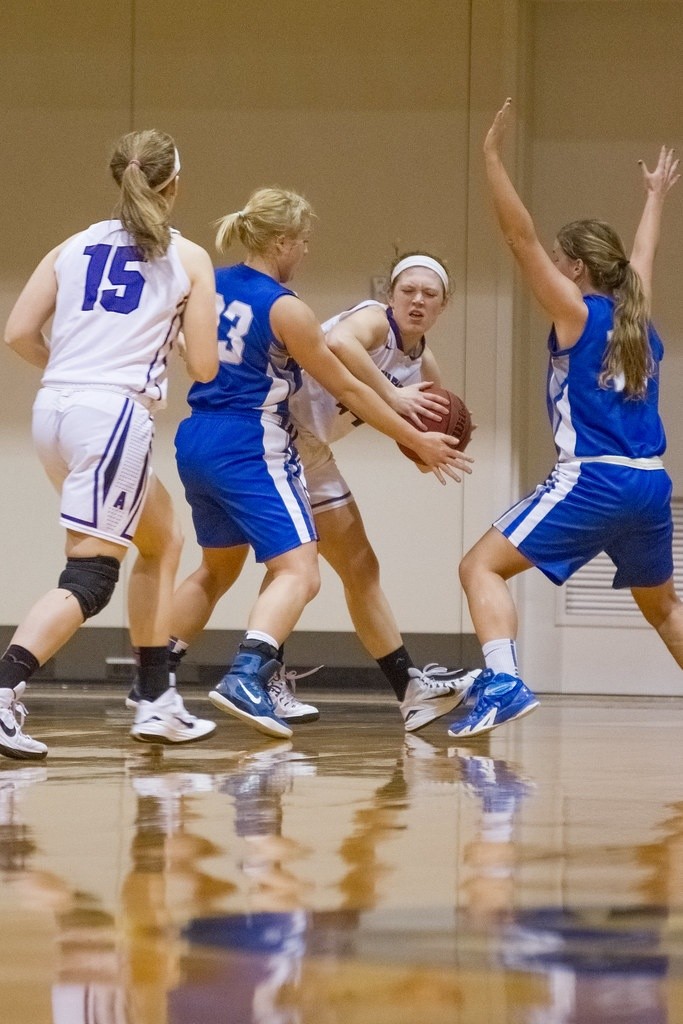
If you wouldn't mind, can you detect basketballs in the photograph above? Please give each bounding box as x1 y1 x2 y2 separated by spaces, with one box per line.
395 387 473 466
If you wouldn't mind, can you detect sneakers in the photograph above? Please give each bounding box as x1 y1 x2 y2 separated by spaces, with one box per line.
208 651 294 738
266 643 324 724
128 687 218 744
0 681 49 760
125 648 198 719
396 662 483 732
447 668 542 738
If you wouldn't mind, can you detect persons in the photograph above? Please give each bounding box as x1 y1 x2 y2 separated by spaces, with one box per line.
164 733 466 1024
0 129 219 761
125 190 482 732
448 97 683 737
445 747 683 1024
0 764 211 1024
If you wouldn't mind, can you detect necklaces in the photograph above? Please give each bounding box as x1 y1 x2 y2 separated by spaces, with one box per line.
400 331 420 363
